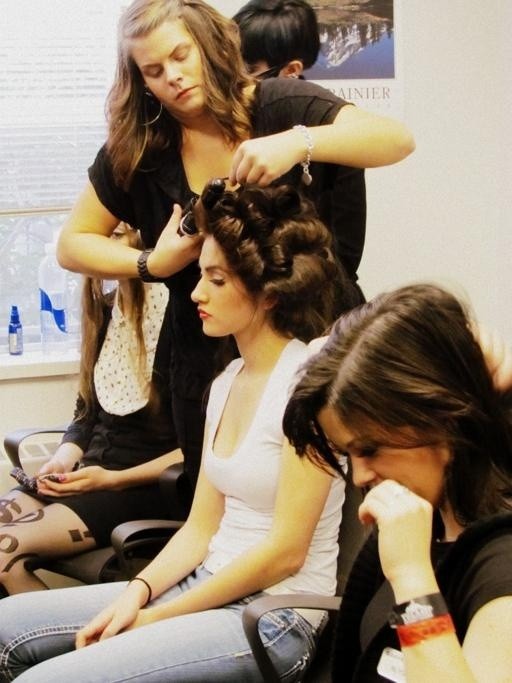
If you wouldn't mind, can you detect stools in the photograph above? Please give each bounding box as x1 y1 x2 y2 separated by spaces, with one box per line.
2 424 185 583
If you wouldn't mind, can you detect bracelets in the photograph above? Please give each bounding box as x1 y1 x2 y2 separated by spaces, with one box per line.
392 590 450 626
292 122 315 187
128 577 152 609
137 247 166 283
395 613 456 648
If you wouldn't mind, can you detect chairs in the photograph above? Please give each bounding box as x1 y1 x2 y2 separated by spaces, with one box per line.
110 484 386 681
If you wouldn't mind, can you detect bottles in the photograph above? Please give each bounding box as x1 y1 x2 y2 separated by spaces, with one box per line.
38 243 68 355
8 304 24 355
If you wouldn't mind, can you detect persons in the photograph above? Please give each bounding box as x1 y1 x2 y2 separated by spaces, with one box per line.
0 177 367 682
0 221 222 596
56 0 420 518
225 0 319 82
280 279 512 679
